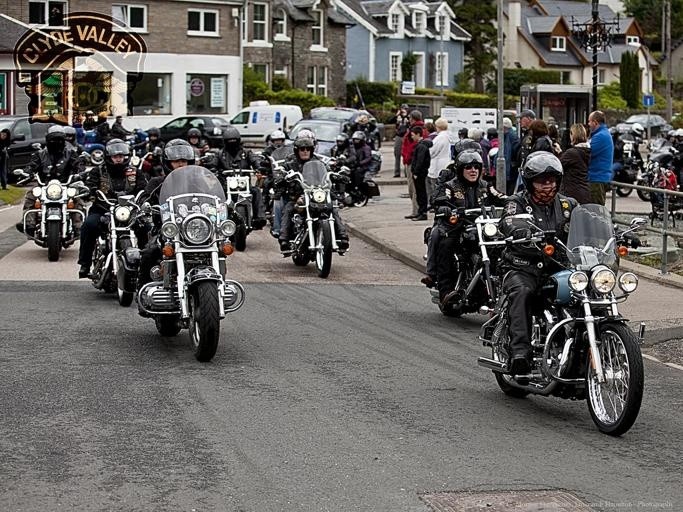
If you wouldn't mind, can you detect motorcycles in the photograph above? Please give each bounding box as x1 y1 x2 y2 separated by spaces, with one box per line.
12 166 93 262
480 203 647 437
30 142 221 170
118 165 245 363
404 198 517 318
67 179 146 307
272 160 351 278
608 124 683 201
223 151 382 250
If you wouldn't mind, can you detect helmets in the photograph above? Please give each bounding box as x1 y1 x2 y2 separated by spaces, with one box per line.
187 128 201 146
293 138 315 159
667 129 677 137
104 138 131 173
335 133 350 148
223 127 241 147
163 138 195 176
85 110 94 116
98 111 108 119
630 122 645 136
148 127 162 143
454 138 484 157
453 148 484 176
521 150 564 196
675 128 683 144
45 124 66 148
351 130 367 146
297 129 317 147
270 131 286 144
64 126 77 142
608 126 622 136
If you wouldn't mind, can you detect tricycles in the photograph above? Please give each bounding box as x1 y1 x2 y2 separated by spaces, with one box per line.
78 129 148 166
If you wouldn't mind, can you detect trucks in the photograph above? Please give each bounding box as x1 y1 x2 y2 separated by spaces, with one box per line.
440 108 517 129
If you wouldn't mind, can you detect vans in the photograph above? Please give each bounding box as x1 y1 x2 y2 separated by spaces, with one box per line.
229 101 304 147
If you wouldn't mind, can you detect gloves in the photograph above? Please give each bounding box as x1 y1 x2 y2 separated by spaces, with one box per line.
129 209 152 233
618 230 642 250
511 223 537 247
231 209 246 228
337 174 351 185
259 166 270 176
273 175 288 190
18 168 34 185
437 204 452 219
79 187 92 201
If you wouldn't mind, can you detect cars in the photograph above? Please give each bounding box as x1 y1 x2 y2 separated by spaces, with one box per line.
609 114 673 138
0 115 54 173
282 107 386 154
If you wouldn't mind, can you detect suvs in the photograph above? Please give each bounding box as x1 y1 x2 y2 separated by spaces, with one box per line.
160 116 240 151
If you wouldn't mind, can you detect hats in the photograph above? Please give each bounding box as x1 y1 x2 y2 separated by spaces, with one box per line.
514 109 537 118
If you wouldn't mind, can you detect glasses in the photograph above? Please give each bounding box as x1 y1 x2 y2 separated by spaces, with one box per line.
464 163 481 170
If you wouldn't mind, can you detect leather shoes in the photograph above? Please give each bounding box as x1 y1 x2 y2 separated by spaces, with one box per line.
338 239 350 255
16 221 36 235
280 241 292 257
420 276 434 288
412 214 428 221
509 353 532 386
404 212 421 219
439 290 459 314
79 264 90 277
252 218 267 230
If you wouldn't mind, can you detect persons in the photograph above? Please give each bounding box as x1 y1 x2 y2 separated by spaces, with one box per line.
393 110 682 287
498 151 620 385
438 151 508 310
1 110 382 287
